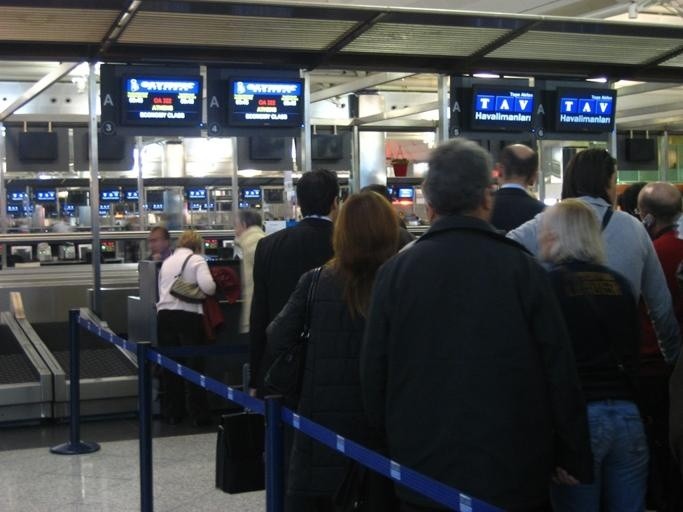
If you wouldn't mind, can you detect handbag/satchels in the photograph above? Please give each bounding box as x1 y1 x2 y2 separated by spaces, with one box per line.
215 409 268 495
619 353 669 414
169 275 206 304
263 334 307 394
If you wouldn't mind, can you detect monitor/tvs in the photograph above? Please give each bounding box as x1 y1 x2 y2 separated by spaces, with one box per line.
100 190 120 201
68 204 76 211
240 202 249 208
554 86 617 133
188 189 207 200
190 204 199 209
227 77 304 128
100 203 110 210
9 192 25 201
120 73 202 127
36 190 56 201
399 188 414 198
7 204 19 212
203 204 214 209
100 210 106 215
152 202 162 210
243 188 261 199
126 189 138 200
470 84 539 131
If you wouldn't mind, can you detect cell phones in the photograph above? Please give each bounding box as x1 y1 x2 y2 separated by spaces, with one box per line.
642 213 657 229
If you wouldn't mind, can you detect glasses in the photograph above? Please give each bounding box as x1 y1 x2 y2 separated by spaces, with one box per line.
634 208 642 214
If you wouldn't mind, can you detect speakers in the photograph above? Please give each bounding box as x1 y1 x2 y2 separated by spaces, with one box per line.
311 134 345 160
18 132 58 161
249 136 286 160
87 132 125 160
626 139 655 162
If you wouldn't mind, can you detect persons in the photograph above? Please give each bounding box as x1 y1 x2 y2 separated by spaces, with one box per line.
637 182 683 511
535 198 651 512
490 144 548 234
145 226 175 261
359 137 595 512
235 211 267 334
247 170 341 512
156 230 217 428
258 191 399 512
503 148 681 366
